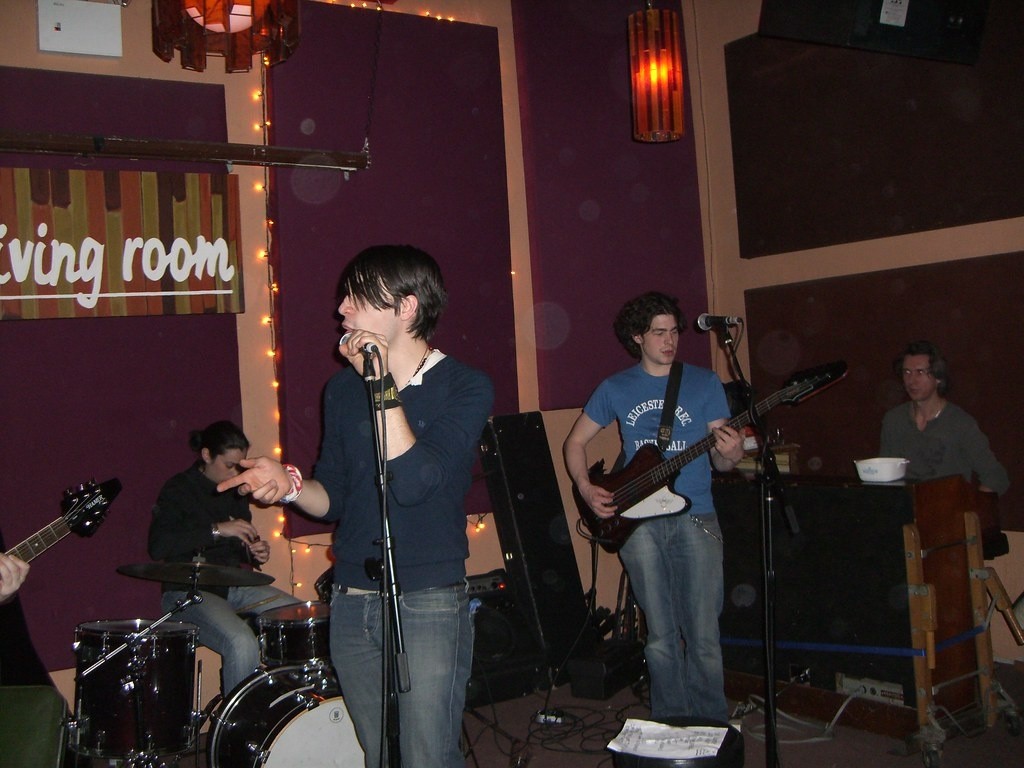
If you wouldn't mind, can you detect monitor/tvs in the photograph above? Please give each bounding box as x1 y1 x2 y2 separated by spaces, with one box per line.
757 0 987 65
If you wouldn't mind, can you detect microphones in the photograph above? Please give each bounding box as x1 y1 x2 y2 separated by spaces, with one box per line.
339 332 378 354
697 313 743 330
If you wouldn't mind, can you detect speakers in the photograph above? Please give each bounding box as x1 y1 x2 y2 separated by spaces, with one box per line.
462 568 546 708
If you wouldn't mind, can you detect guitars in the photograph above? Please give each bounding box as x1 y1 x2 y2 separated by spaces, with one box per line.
3 474 125 564
578 360 849 554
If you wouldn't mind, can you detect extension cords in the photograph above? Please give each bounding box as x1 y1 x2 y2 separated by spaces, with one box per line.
535 713 562 724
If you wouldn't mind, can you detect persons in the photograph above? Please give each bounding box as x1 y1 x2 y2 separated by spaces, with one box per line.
878 340 1010 495
0 533 89 768
563 293 745 725
216 244 493 768
148 421 303 701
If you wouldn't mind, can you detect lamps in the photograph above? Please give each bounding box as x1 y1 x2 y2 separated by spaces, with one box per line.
149 0 303 76
629 3 687 144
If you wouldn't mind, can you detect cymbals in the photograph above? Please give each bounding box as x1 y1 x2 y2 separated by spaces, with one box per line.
116 562 277 586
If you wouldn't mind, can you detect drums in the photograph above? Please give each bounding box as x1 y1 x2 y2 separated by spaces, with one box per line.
205 664 365 768
69 619 200 759
256 601 333 666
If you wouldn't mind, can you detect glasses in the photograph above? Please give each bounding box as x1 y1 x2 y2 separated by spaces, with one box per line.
901 368 930 377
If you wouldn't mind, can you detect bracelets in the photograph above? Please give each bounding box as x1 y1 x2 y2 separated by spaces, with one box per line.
279 464 302 504
211 523 220 541
411 347 433 377
934 410 941 418
373 374 401 411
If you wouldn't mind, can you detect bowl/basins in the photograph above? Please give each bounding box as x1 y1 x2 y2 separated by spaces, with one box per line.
855 458 910 482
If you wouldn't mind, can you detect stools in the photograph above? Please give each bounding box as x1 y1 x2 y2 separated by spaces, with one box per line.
174 617 263 759
613 715 746 768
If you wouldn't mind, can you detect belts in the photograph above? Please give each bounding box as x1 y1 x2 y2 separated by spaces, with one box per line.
336 582 464 595
